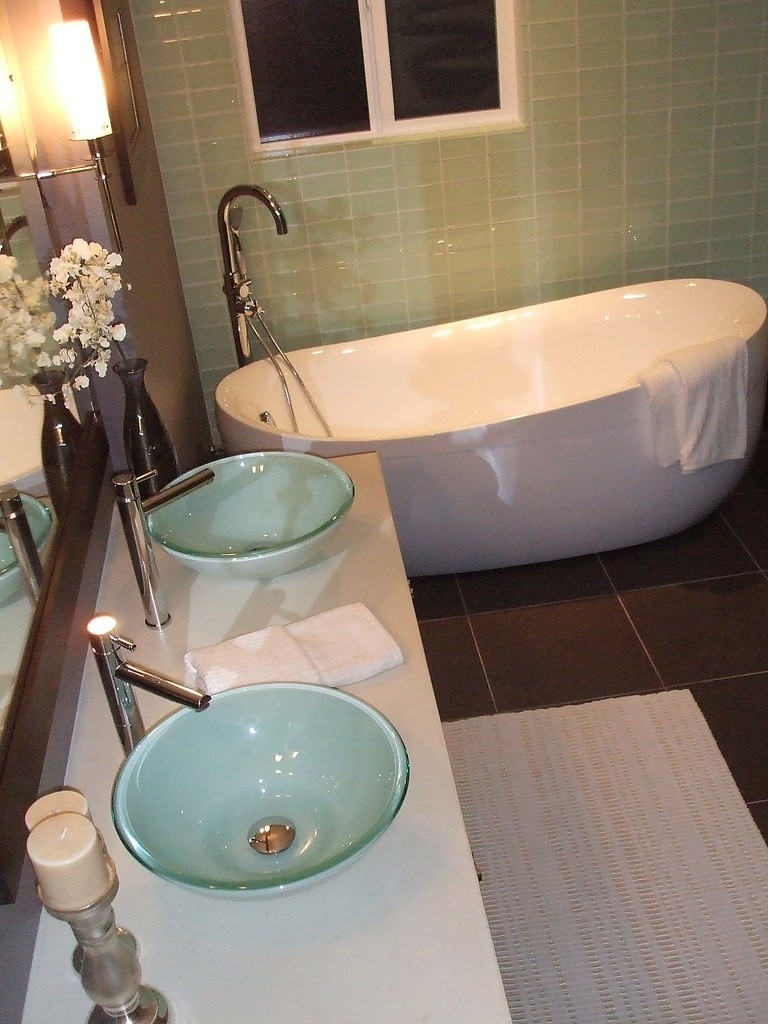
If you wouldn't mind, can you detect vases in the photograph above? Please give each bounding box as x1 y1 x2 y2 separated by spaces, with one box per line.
29 368 82 519
112 357 183 503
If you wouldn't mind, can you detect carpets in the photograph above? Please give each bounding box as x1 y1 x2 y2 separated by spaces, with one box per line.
443 688 768 1023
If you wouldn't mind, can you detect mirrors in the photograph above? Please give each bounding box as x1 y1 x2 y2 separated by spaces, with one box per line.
0 37 112 909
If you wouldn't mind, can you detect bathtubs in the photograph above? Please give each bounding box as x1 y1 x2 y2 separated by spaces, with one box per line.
212 275 767 581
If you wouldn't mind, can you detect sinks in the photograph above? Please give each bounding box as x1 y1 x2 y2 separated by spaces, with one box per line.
146 451 356 582
1 491 53 602
109 681 403 901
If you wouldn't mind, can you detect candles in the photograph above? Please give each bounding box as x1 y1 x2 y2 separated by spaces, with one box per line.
25 791 93 832
26 810 117 910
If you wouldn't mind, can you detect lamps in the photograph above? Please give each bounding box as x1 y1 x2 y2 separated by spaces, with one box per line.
37 20 125 253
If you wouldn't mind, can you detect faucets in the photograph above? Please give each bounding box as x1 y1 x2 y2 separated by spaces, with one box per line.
0 488 45 602
85 615 212 760
215 182 288 368
111 468 216 626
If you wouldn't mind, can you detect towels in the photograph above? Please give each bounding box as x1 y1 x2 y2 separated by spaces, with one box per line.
626 334 749 477
184 601 405 696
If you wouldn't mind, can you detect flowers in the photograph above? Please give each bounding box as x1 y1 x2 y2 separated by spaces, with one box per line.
0 244 90 409
49 238 132 379
